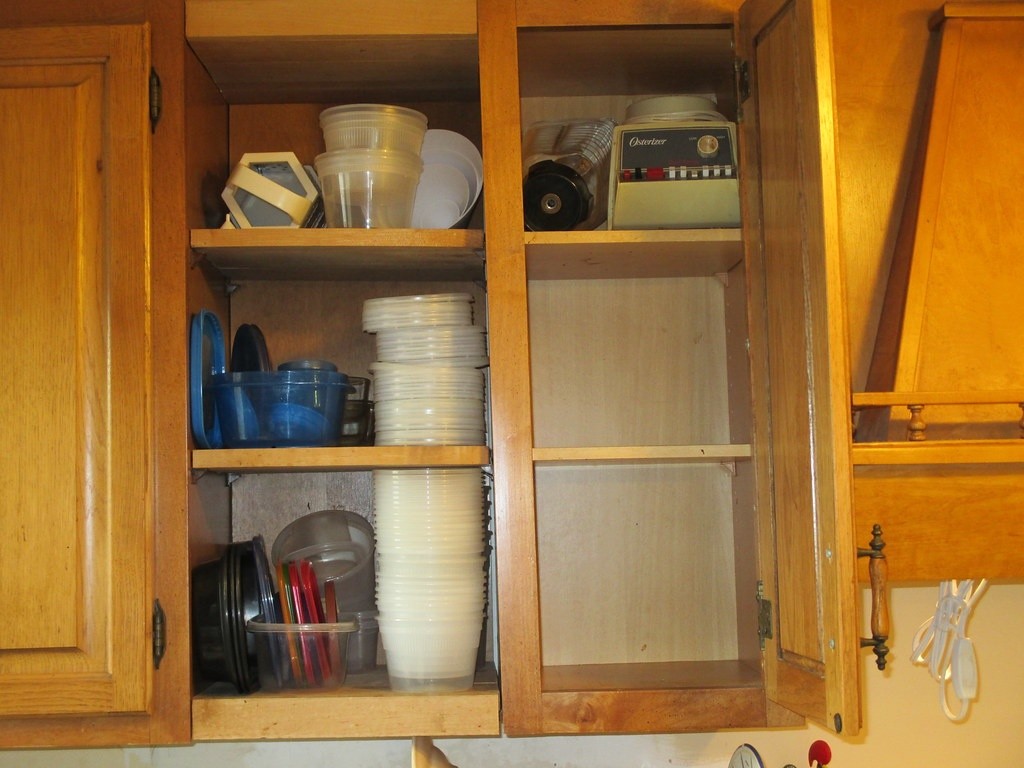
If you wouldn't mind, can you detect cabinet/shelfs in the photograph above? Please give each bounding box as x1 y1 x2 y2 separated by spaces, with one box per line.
0 0 891 746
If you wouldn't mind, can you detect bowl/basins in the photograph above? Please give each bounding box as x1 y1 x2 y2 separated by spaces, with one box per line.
314 102 483 232
213 370 352 450
372 468 491 693
252 506 378 692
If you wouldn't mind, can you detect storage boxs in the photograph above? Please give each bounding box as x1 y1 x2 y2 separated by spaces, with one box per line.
246 612 360 692
